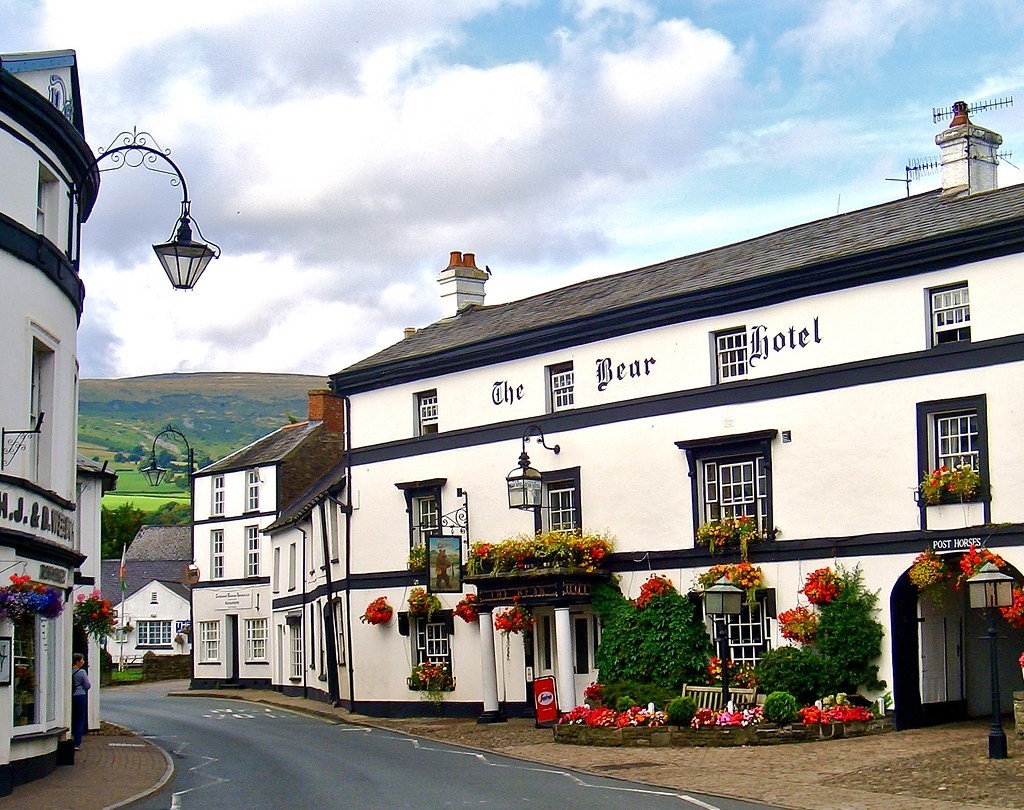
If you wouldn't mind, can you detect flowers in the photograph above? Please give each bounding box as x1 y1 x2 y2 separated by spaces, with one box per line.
413 661 451 712
798 567 840 604
630 572 673 608
907 546 952 612
0 573 65 625
955 544 1023 630
73 588 118 641
777 606 818 646
694 513 768 562
695 562 764 606
174 634 184 653
466 526 614 575
919 464 981 504
123 623 134 632
359 587 540 660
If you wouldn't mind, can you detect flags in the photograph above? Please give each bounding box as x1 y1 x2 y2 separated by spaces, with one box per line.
118 544 128 592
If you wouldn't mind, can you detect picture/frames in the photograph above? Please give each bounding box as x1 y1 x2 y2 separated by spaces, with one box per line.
0 636 12 687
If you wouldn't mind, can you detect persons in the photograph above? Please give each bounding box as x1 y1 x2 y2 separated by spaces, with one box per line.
72 653 91 751
14 665 35 725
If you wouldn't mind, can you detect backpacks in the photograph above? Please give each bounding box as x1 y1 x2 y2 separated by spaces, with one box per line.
71 668 82 693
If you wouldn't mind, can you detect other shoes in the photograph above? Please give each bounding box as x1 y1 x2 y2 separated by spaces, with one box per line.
74 746 82 750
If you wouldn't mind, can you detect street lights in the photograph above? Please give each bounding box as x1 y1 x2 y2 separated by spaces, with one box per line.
702 575 743 713
968 560 1013 759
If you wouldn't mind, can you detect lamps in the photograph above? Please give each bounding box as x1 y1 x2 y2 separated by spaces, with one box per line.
73 124 222 292
140 429 190 487
506 424 556 510
